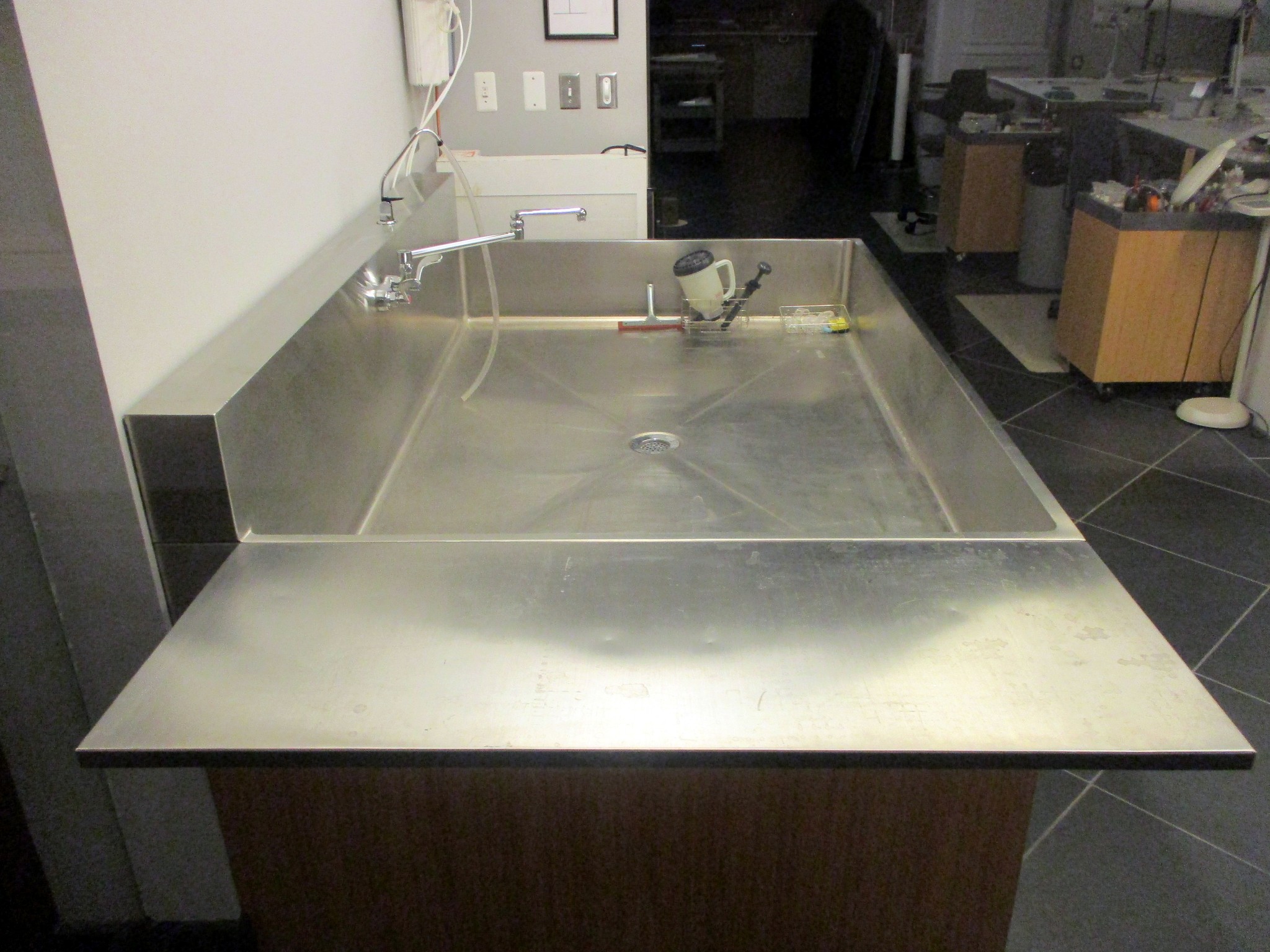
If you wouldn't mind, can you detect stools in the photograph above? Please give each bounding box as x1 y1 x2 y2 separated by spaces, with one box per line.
649 58 726 153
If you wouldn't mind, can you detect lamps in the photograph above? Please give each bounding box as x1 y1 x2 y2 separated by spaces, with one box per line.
1169 123 1270 430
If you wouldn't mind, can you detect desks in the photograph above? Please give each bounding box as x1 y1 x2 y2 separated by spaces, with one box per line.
989 76 1270 186
649 11 819 120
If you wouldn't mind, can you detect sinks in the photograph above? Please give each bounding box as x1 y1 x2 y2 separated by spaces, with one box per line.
214 238 1084 543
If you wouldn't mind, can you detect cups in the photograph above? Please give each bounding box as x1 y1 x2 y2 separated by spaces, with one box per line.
672 250 735 319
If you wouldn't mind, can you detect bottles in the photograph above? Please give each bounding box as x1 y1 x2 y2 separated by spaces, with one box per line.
1125 179 1171 214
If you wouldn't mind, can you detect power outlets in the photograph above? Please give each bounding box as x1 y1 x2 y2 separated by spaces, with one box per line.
474 71 498 112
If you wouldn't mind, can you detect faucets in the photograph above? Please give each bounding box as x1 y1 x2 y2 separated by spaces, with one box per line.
376 208 586 324
377 129 443 225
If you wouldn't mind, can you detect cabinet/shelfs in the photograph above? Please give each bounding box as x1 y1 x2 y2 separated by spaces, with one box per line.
1050 187 1265 403
934 121 1056 267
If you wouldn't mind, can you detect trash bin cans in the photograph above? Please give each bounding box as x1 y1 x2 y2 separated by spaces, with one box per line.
1018 138 1072 287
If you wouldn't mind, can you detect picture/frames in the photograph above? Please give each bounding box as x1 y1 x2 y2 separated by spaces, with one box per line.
543 0 619 40
